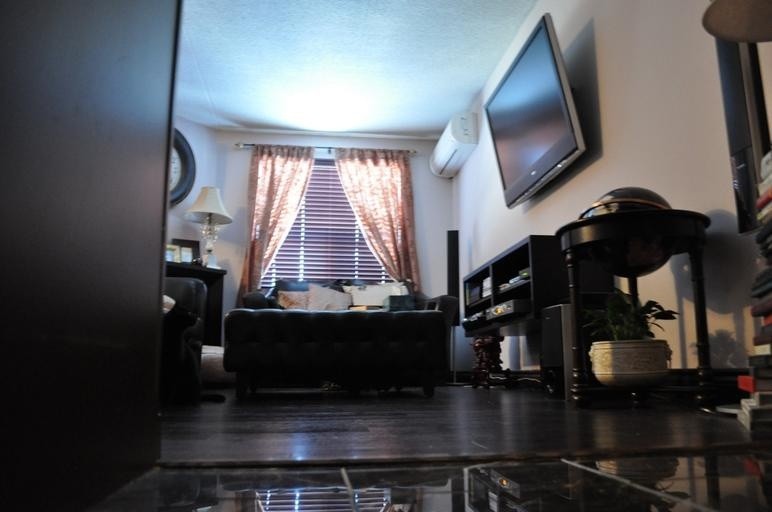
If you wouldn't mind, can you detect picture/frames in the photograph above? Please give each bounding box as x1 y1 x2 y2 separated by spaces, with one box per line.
169 127 196 208
166 244 181 263
172 239 201 266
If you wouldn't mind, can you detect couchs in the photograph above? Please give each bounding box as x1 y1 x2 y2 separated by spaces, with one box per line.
222 308 446 398
242 285 434 325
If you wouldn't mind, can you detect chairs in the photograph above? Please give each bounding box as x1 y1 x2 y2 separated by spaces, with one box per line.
419 296 458 331
162 278 208 422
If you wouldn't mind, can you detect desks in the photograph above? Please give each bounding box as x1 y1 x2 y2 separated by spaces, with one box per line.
464 303 583 401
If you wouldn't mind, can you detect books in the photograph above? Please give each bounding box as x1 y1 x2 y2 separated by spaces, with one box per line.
731 148 772 435
716 404 740 414
347 304 384 311
469 273 530 304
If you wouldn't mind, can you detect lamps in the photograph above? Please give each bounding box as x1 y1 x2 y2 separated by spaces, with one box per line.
185 186 233 267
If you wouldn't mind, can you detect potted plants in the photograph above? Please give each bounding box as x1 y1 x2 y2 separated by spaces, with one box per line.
573 287 682 385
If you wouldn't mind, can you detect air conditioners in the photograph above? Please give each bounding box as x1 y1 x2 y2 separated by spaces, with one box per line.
429 111 477 178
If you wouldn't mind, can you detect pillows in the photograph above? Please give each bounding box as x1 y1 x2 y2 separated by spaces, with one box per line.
276 280 409 310
162 294 176 315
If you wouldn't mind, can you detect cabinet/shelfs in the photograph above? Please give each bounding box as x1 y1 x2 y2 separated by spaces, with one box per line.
462 234 574 332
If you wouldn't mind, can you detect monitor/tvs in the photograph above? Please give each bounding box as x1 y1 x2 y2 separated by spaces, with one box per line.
483 13 586 209
